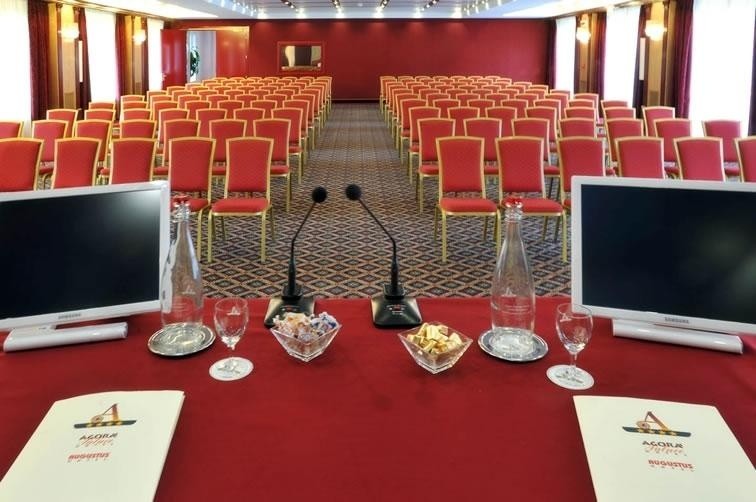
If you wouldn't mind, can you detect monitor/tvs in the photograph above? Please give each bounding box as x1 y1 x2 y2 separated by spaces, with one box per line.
571 174 755 356
0 180 172 353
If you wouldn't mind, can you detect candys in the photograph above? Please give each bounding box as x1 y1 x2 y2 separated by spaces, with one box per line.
405 322 464 358
271 308 338 344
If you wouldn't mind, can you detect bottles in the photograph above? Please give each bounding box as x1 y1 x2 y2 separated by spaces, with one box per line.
488 198 537 356
157 199 204 349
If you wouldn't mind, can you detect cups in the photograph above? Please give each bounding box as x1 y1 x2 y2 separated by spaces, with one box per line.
210 295 251 379
550 301 595 388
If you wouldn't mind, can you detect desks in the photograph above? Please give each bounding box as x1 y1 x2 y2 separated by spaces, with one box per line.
0 298 756 502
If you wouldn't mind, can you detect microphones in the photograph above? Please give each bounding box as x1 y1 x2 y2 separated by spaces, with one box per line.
346 184 423 330
263 185 327 330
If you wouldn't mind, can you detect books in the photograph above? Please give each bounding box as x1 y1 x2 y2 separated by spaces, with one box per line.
573 393 756 502
0 390 185 502
